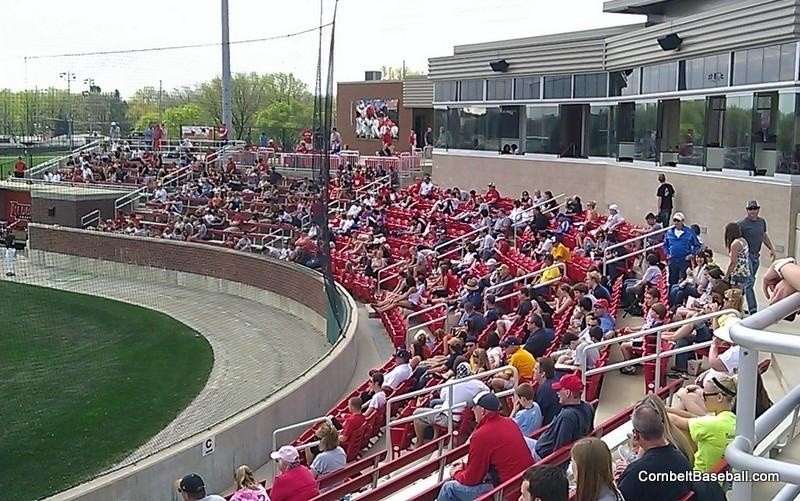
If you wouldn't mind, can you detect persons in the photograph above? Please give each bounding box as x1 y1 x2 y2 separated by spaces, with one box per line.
12 155 27 178
355 99 398 137
3 226 18 278
44 120 799 501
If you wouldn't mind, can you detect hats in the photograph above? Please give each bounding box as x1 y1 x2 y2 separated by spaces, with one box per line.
269 181 761 500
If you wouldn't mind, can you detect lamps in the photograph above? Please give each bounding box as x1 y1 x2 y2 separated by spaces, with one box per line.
490 58 509 72
657 32 683 51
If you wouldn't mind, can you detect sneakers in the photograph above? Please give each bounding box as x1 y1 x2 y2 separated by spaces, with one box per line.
6 271 15 276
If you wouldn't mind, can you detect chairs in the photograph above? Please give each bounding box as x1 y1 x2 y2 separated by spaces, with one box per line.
46 142 330 263
